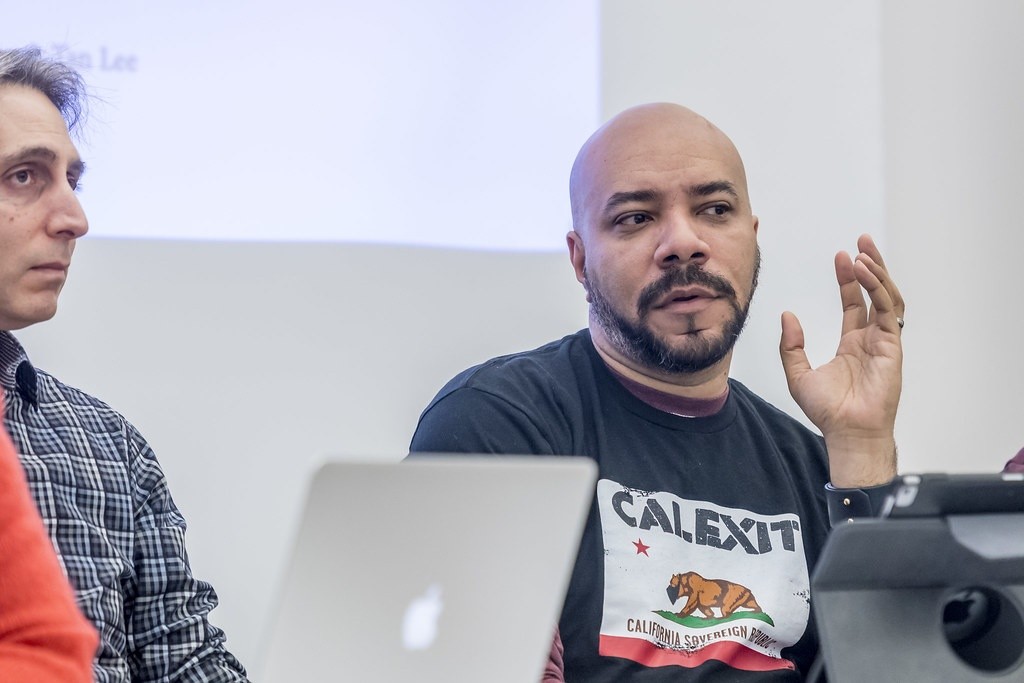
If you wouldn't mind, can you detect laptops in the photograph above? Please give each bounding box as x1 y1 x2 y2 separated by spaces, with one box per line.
258 457 601 683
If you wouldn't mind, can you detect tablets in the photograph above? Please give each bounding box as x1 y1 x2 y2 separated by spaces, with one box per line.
805 472 1023 683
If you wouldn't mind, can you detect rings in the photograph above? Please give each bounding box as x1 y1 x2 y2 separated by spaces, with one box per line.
896 317 904 328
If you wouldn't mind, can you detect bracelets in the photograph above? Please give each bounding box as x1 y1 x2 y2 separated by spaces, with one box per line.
824 475 899 530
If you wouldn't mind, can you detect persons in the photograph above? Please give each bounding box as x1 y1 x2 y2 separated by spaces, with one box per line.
0 43 248 683
405 102 905 683
